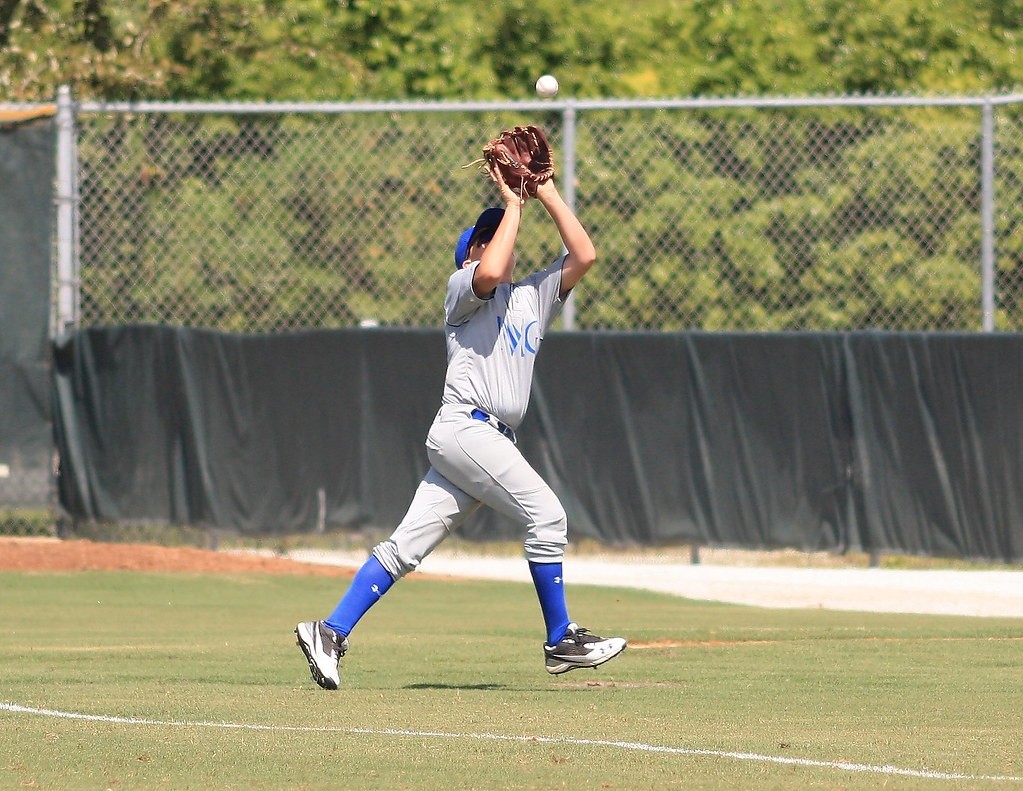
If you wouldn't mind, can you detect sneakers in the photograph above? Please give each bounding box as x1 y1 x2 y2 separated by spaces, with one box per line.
295 619 350 690
543 623 628 673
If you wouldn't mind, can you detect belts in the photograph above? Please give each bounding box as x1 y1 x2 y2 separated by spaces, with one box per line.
471 408 516 442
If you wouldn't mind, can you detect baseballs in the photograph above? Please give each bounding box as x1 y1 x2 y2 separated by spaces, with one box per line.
533 73 560 99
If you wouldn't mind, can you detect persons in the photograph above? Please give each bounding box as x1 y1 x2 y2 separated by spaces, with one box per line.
296 125 628 693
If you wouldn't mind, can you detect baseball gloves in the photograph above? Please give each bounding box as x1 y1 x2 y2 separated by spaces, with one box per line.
459 123 558 206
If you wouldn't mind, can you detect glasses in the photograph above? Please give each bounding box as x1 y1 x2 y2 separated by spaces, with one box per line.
469 227 493 245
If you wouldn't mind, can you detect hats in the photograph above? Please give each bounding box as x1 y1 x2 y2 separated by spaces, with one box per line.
454 207 504 270
